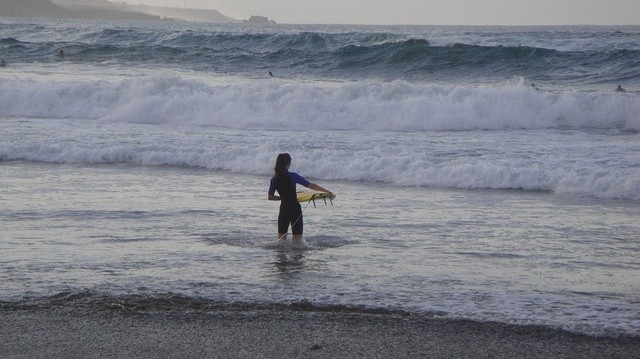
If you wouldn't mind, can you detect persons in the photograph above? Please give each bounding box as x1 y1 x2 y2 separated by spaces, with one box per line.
268 153 331 244
56 50 63 54
613 84 625 92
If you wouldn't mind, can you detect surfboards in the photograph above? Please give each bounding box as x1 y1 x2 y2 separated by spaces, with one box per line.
295 192 336 203
